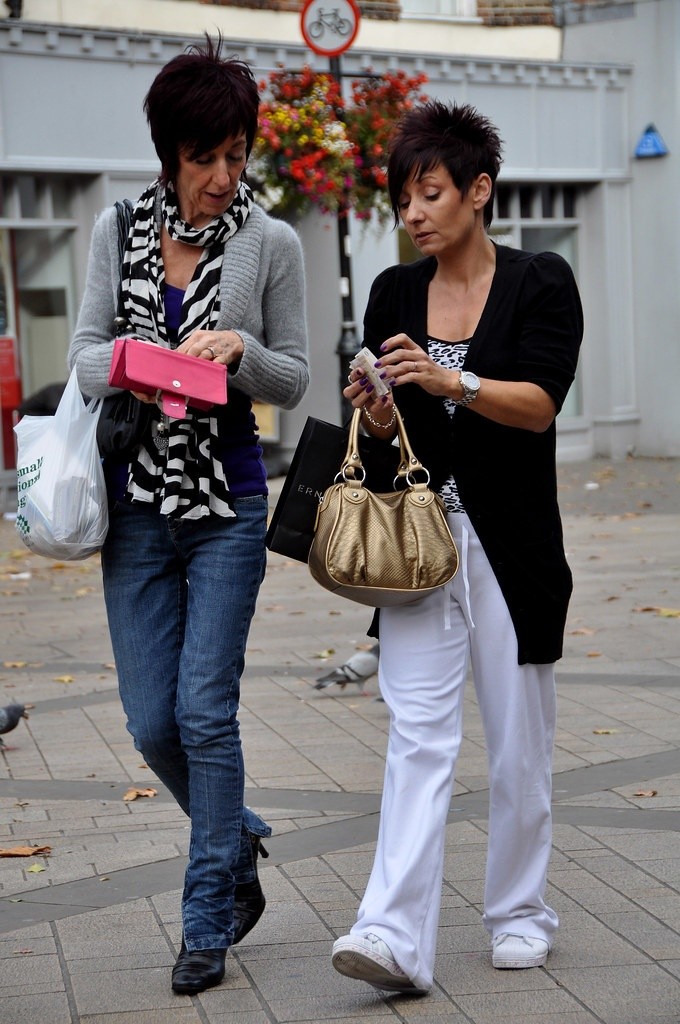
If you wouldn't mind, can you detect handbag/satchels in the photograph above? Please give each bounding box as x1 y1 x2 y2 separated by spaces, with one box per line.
263 405 413 564
13 363 109 560
308 388 459 608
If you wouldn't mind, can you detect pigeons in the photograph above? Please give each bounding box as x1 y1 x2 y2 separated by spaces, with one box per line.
0 703 29 750
314 643 380 695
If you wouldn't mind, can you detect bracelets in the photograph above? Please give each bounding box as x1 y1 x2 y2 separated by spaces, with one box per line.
364 404 397 429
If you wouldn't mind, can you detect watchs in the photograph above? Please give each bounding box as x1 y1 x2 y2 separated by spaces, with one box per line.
453 372 480 405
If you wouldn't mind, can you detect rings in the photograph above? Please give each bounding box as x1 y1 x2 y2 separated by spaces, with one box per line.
348 376 353 384
415 361 418 369
207 347 215 358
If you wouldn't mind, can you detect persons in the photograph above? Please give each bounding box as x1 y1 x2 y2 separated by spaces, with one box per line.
332 109 585 992
69 51 311 994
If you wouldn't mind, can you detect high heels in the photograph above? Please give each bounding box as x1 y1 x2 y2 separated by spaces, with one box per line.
228 824 269 944
172 937 228 994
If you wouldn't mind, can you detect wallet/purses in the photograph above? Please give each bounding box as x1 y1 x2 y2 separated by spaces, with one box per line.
108 339 227 420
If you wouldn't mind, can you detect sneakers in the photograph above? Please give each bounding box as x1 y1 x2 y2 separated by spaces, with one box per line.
492 931 549 969
332 932 432 998
